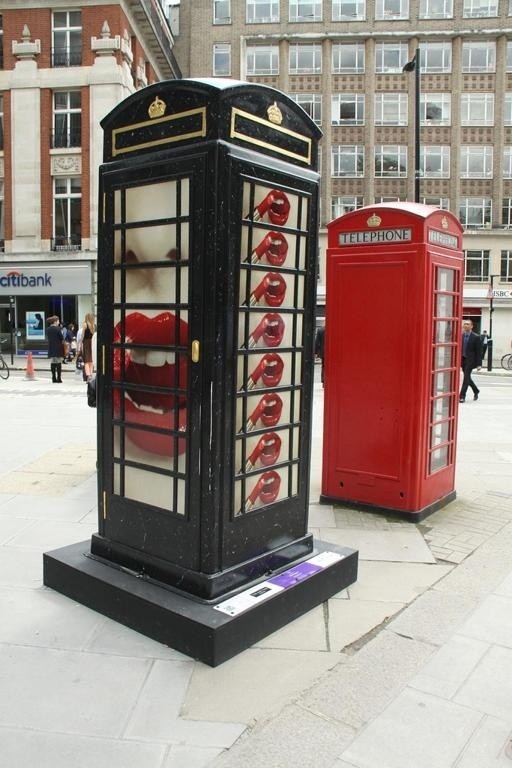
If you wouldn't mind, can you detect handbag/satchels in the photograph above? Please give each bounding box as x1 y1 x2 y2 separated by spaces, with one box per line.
76 357 84 370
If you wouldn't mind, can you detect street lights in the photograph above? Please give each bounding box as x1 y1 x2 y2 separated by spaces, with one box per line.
9 295 14 364
403 47 420 202
488 272 500 370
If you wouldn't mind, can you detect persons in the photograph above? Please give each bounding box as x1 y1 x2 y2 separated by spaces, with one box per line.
235 177 311 517
78 313 95 382
32 313 43 330
46 316 66 383
67 324 77 361
112 178 189 518
58 321 68 363
315 323 326 387
458 320 480 403
475 329 489 370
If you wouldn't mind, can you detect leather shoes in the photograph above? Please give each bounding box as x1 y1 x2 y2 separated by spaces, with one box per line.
474 390 479 400
460 399 464 402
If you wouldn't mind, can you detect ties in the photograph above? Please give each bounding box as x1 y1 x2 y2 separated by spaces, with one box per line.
463 335 467 353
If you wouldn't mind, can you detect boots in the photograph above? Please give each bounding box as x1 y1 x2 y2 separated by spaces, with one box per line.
51 362 62 382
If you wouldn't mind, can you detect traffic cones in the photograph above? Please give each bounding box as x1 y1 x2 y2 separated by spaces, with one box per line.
23 352 36 381
